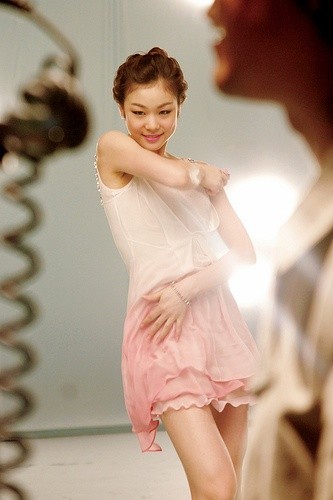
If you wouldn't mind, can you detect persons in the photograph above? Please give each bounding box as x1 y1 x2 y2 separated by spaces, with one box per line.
206 0 333 500
94 46 261 500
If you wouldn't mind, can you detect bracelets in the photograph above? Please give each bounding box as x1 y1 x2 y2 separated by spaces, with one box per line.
170 281 192 305
